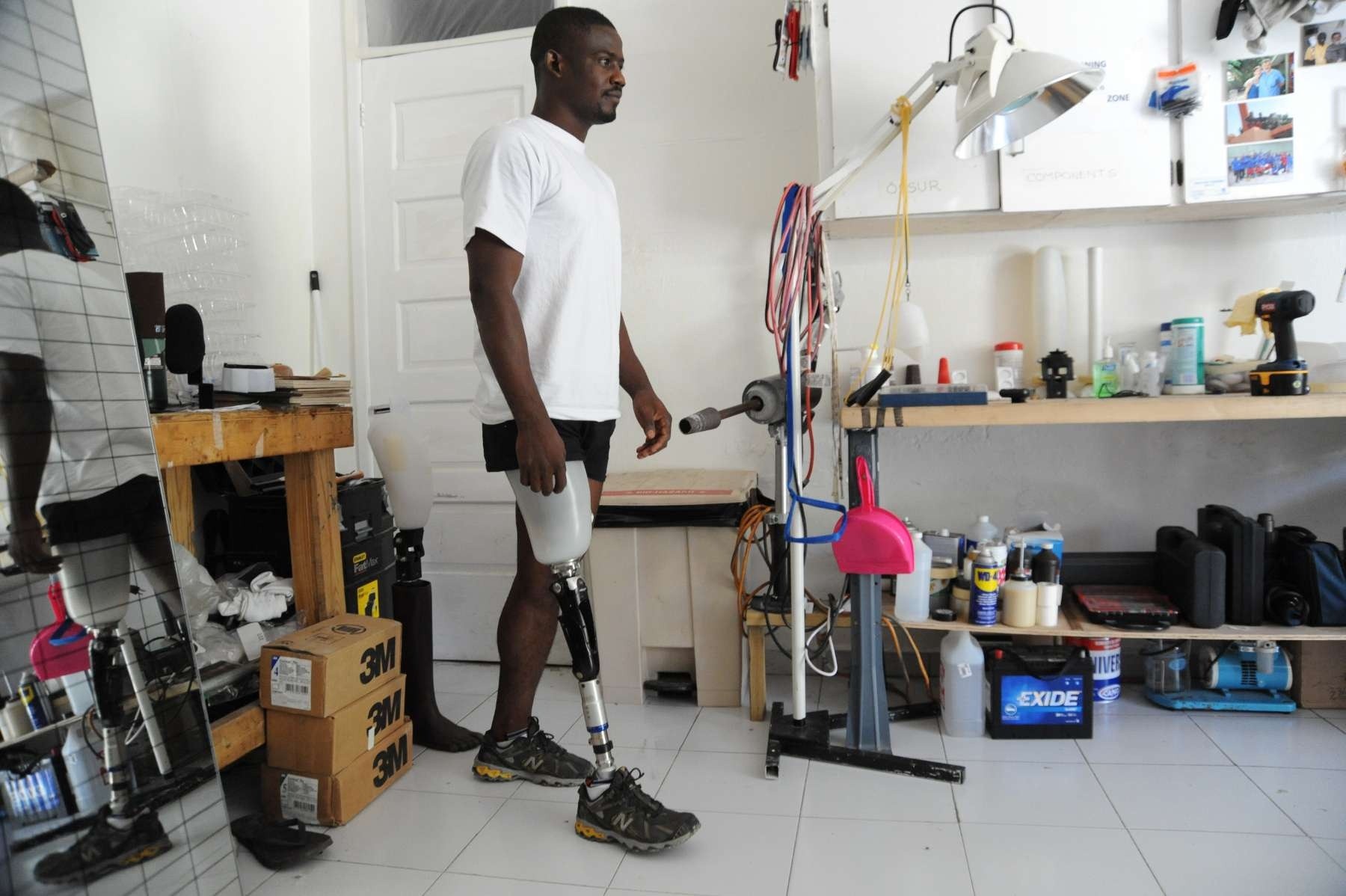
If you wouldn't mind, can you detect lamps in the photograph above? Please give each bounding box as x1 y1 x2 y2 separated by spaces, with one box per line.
766 0 1104 781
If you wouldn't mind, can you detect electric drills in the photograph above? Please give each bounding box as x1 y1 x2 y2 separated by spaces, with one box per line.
1220 289 1315 396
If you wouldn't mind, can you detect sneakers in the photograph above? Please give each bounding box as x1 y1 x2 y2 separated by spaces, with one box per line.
574 763 702 852
34 800 176 886
472 716 596 788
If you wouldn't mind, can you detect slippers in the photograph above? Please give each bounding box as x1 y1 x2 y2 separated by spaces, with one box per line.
230 809 332 869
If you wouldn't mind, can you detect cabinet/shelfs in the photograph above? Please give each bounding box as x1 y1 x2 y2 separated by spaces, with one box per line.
1 672 198 848
804 0 1343 235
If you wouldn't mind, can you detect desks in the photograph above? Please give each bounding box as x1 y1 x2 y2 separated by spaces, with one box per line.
152 404 353 768
844 387 1346 751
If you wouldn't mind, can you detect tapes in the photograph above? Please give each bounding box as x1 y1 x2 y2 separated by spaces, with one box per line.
221 365 277 393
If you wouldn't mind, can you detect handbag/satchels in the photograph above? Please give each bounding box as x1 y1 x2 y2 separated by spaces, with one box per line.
1273 524 1346 626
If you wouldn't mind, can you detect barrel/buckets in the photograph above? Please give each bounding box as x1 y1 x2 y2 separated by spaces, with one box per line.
939 631 987 738
61 718 137 813
1063 636 1121 704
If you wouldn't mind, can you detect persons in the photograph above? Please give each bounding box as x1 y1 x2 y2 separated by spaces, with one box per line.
462 6 700 854
0 176 208 884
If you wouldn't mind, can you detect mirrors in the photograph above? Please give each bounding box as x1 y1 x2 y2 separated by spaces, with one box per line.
1 1 244 896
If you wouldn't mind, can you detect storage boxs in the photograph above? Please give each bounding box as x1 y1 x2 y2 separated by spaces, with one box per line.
261 613 412 830
1287 640 1346 709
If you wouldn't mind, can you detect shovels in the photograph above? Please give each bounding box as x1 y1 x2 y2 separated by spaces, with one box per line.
832 456 915 574
30 582 92 682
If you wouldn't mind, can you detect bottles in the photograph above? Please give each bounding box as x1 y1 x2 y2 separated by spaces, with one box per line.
18 672 56 730
1160 317 1206 394
995 340 1024 392
892 512 1063 628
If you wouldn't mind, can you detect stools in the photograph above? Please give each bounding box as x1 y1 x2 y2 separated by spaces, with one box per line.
746 596 852 720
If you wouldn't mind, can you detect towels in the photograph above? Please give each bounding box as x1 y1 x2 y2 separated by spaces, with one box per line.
217 571 294 624
